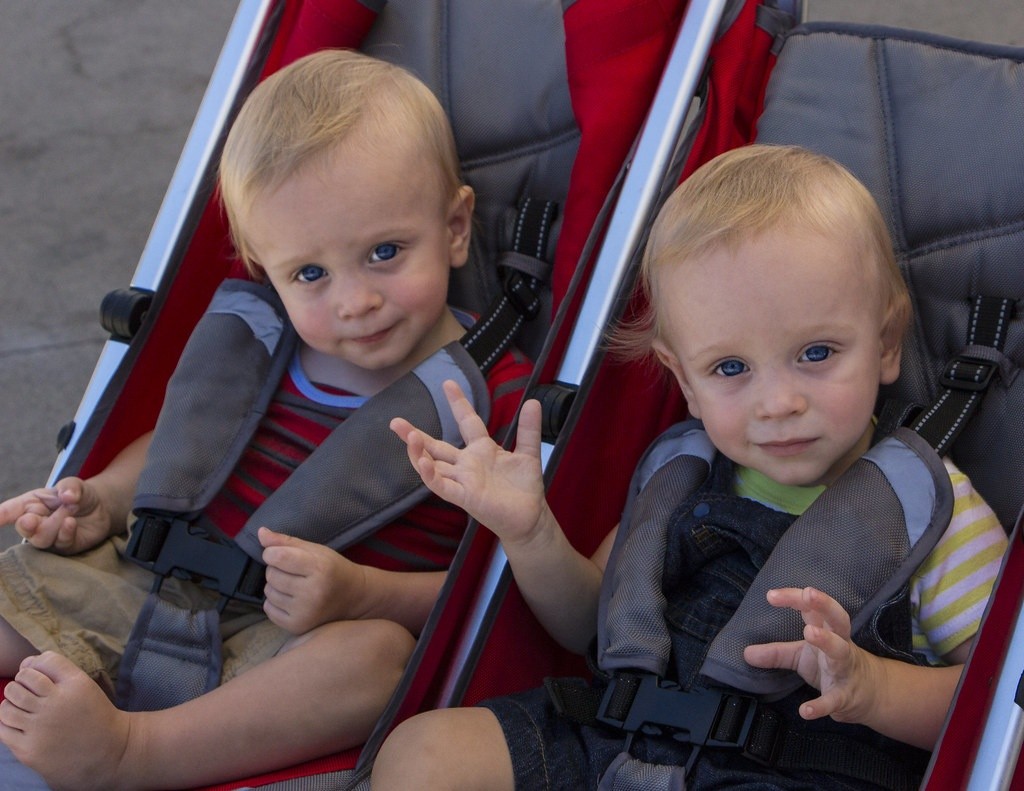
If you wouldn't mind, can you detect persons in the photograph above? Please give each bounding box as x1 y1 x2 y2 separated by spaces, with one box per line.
0 51 544 791
373 145 1015 791
